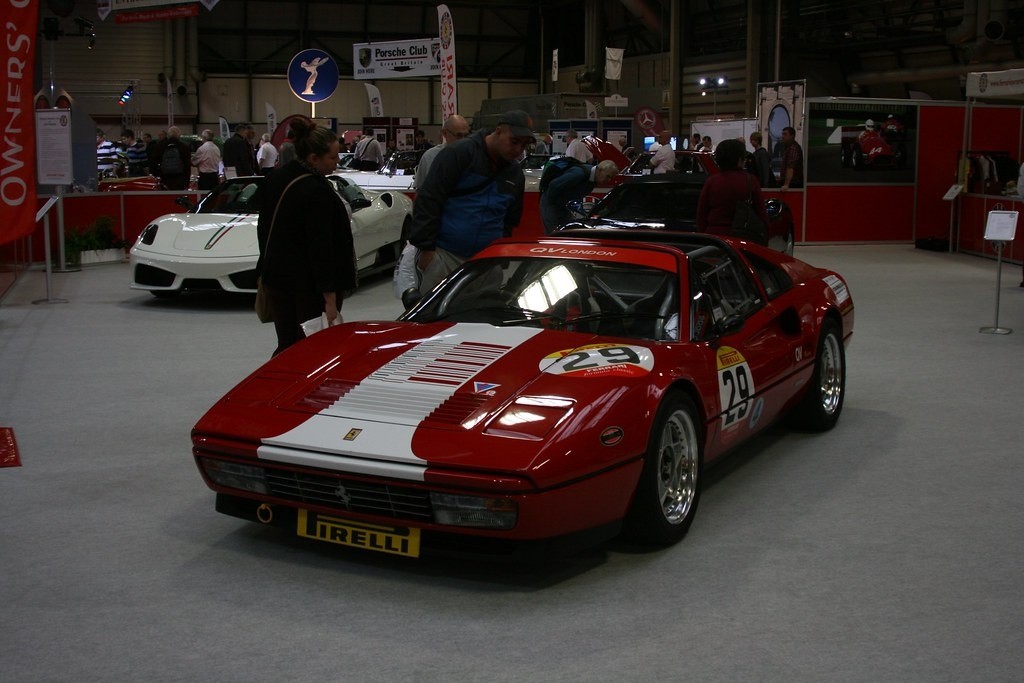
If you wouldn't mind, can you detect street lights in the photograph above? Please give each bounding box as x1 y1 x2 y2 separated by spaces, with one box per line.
699 77 730 119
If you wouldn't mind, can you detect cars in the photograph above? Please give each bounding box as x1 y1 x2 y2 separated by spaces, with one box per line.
98 134 631 191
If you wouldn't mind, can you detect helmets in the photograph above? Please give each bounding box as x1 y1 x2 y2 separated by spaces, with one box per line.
865 119 875 131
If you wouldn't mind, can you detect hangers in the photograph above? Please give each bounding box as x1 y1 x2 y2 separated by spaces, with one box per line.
960 152 993 163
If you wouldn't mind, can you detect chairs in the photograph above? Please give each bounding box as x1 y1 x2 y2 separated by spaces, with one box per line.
509 261 601 338
239 184 257 203
622 261 725 344
607 192 646 217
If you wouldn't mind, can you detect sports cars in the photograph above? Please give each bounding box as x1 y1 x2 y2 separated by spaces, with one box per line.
189 226 856 566
128 174 415 302
552 148 795 260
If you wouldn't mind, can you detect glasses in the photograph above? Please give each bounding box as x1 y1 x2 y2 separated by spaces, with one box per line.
446 129 473 139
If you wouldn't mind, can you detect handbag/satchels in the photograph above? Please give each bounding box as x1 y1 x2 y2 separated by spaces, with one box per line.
392 240 421 301
729 173 769 245
255 285 278 323
769 168 777 188
352 158 361 169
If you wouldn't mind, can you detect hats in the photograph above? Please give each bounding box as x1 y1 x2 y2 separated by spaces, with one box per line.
261 133 272 142
497 110 537 146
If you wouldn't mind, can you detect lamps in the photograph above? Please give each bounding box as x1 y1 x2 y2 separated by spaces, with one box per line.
87 38 95 49
73 16 94 30
699 76 725 96
118 87 132 104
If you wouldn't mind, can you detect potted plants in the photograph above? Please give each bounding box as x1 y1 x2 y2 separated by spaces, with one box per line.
50 216 134 264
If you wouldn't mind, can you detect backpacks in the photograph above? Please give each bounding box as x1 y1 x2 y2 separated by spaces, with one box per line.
161 140 182 175
539 156 590 193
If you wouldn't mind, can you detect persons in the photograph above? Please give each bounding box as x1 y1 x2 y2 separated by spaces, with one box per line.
565 129 593 164
699 136 716 152
408 109 537 297
737 137 752 173
539 160 619 234
338 128 397 171
780 127 803 192
688 133 700 151
649 136 662 152
747 132 771 188
535 135 553 155
95 124 295 191
619 137 633 158
858 119 879 139
415 114 469 188
257 119 358 359
887 114 896 126
695 139 766 246
414 131 434 150
649 131 675 174
